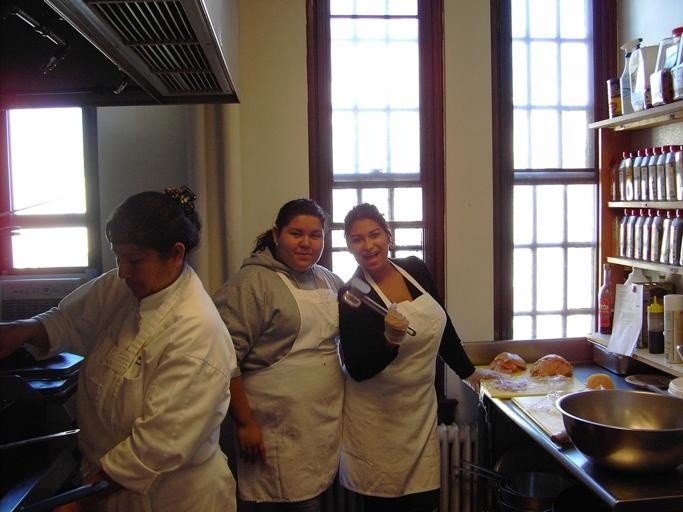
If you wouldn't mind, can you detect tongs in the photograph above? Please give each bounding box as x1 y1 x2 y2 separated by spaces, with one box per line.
340 277 417 337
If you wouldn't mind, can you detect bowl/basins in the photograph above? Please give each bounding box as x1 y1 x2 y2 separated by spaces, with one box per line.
555 388 683 472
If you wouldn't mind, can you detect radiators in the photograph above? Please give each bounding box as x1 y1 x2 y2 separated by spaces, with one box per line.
439 421 478 512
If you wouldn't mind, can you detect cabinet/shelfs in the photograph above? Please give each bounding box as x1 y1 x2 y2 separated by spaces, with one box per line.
588 99 683 379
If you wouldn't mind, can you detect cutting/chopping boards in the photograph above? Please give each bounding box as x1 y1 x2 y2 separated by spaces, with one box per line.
473 363 590 437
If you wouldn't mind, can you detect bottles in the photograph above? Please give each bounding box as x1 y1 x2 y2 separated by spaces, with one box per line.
599 263 617 335
610 144 683 201
620 209 683 266
663 294 683 364
647 295 664 354
622 267 648 348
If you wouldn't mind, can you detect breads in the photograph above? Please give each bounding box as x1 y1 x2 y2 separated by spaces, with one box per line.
529 354 572 377
489 352 528 374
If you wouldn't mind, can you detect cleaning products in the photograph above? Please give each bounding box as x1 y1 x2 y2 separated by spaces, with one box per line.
621 37 646 112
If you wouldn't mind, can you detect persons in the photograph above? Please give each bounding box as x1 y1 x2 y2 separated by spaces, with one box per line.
337 202 499 512
0 184 239 512
211 198 345 512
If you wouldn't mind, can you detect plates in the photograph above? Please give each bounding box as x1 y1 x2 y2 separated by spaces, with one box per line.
624 374 672 388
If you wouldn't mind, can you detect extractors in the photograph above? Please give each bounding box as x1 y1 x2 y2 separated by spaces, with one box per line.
0 1 242 111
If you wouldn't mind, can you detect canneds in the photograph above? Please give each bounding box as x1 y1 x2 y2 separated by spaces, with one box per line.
606 78 622 119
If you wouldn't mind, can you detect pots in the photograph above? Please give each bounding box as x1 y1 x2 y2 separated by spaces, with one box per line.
451 459 566 512
